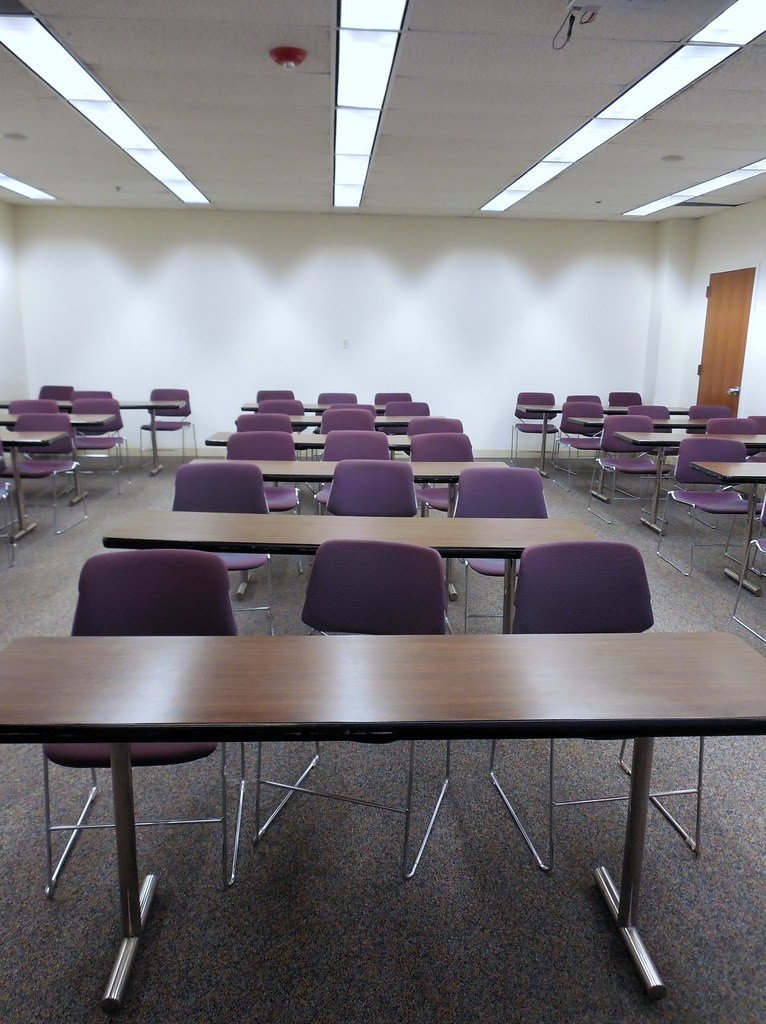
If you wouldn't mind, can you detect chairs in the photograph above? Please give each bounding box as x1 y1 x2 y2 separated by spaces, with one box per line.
253 540 450 881
489 542 704 872
0 385 766 636
42 548 247 896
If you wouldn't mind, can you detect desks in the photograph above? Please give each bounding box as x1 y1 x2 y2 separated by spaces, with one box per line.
0 432 69 543
234 415 442 426
0 637 766 1015
517 405 690 479
567 417 708 503
240 402 395 461
205 431 430 517
612 431 766 536
189 458 512 602
0 399 186 476
0 413 117 506
103 511 595 635
687 462 765 596
